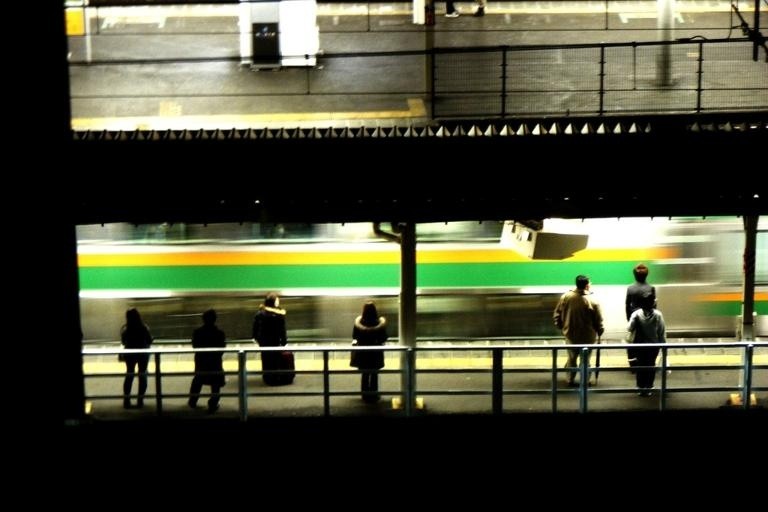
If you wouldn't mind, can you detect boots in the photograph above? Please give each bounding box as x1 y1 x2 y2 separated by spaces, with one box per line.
361 385 381 403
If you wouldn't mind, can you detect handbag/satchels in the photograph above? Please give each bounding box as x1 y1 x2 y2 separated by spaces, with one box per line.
350 339 361 367
623 329 636 343
118 353 126 361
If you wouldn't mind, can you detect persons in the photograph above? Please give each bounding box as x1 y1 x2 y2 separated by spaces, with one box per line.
351 302 388 402
252 293 288 385
187 309 226 411
552 275 600 387
627 291 668 398
625 263 657 374
120 307 152 407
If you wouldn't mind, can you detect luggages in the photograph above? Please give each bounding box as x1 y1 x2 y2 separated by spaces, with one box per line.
261 351 295 386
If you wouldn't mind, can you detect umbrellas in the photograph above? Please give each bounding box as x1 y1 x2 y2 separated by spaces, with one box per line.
594 335 601 382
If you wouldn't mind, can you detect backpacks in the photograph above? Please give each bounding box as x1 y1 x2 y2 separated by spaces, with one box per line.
250 310 275 346
